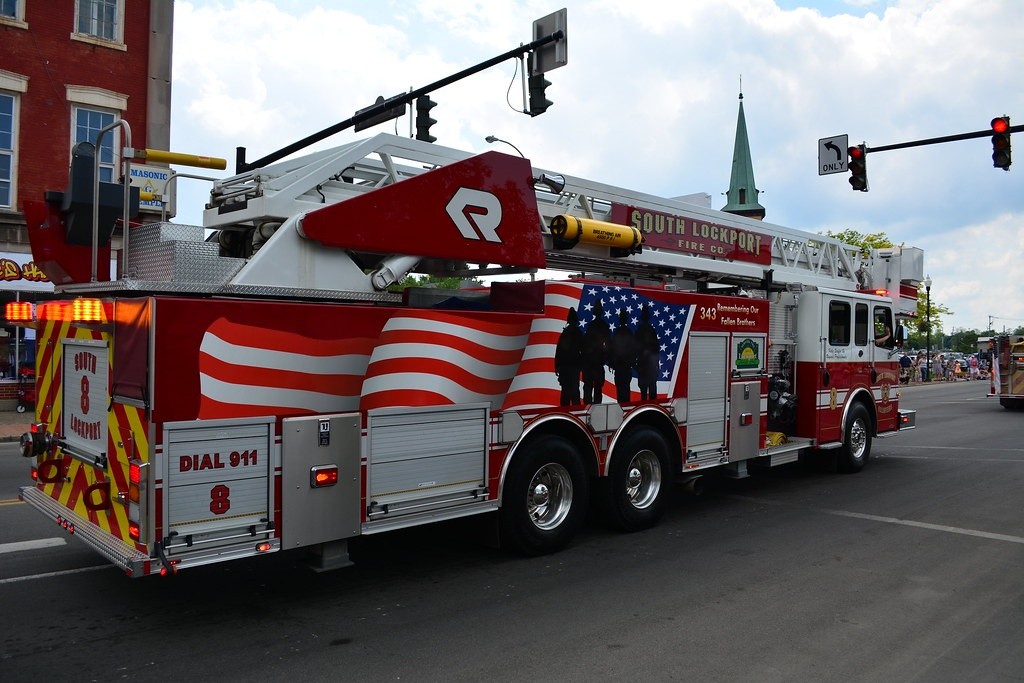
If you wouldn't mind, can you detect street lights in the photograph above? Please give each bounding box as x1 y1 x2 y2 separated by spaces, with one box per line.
924 274 933 381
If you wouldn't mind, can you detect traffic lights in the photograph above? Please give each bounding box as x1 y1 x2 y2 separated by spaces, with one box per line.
846 144 868 190
991 116 1012 168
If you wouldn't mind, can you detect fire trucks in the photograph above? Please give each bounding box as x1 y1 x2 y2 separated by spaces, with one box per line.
986 332 1024 409
5 132 926 581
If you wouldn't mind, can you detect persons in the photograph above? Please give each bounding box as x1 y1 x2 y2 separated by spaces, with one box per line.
873 325 893 346
899 351 989 385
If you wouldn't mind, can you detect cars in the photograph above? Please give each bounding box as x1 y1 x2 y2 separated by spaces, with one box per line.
910 356 933 368
936 353 967 370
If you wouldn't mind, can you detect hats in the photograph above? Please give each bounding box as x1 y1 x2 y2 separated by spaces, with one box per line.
972 355 975 357
